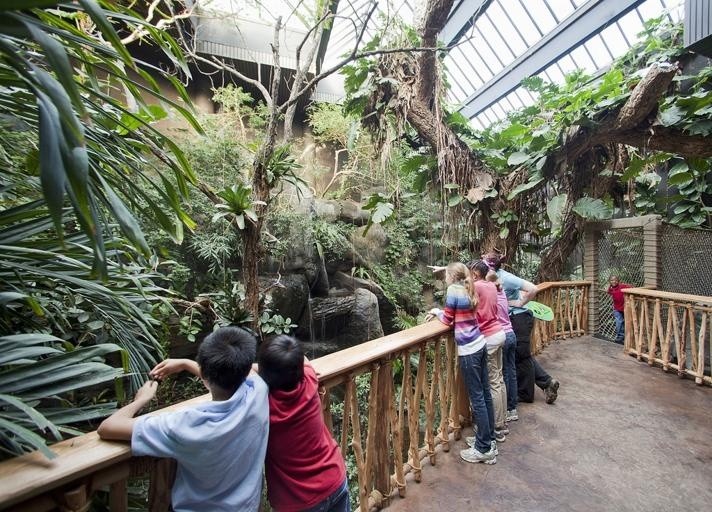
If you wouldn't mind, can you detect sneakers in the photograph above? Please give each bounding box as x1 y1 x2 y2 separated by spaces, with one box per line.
460 409 519 465
544 380 559 404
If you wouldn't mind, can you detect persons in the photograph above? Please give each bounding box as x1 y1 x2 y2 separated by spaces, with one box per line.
97 325 273 511
424 252 559 404
485 267 519 422
599 273 632 344
464 257 510 442
248 332 353 511
424 261 498 466
